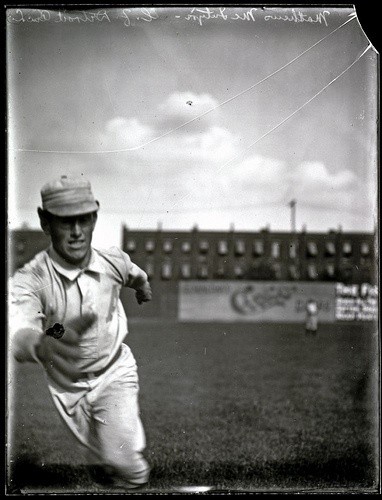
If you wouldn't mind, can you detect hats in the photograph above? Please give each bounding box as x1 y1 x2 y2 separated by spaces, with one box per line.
42 174 98 216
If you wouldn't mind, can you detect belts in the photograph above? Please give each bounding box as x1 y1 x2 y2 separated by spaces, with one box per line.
82 344 122 379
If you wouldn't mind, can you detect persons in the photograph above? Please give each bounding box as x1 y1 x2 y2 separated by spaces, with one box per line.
304 297 319 336
6 173 153 490
